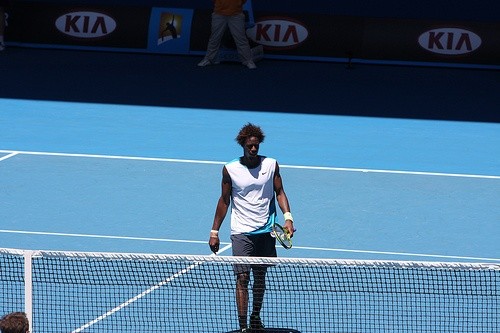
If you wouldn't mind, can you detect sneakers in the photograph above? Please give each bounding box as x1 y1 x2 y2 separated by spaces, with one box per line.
246 63 256 70
197 59 213 67
239 317 264 333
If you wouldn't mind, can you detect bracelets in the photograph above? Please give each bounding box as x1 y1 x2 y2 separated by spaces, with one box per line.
210 230 219 238
283 212 294 223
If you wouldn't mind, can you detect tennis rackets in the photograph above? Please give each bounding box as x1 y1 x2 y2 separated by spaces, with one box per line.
272 223 297 250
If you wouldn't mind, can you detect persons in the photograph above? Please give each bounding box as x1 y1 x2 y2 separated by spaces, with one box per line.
0 312 30 333
209 122 294 333
198 0 256 69
